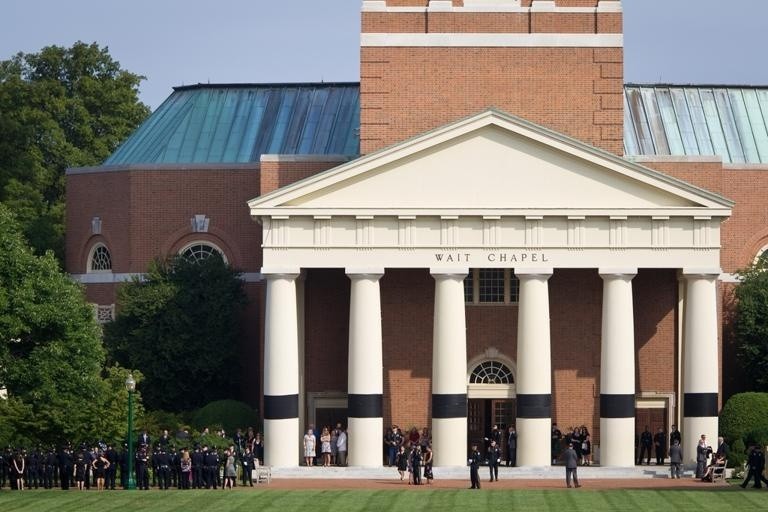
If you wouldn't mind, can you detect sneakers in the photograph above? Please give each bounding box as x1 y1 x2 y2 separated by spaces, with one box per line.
739 483 768 489
401 479 434 486
307 464 347 467
468 486 481 489
567 485 582 488
490 479 498 482
0 485 255 491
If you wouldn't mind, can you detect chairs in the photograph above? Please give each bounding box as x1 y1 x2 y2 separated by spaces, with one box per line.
708 460 727 484
253 458 273 485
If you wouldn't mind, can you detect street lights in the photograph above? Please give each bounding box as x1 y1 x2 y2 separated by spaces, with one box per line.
123 373 138 489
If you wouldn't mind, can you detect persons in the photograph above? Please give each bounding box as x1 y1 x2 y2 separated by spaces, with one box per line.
552 423 590 488
468 424 516 489
635 425 768 488
303 422 347 467
384 425 433 485
0 422 265 491
